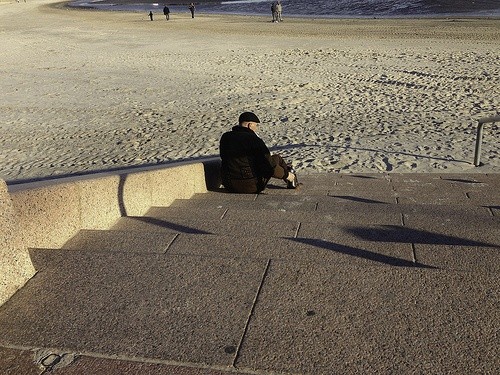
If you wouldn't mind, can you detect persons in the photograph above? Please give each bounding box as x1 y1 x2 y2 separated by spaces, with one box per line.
148 11 154 21
189 2 196 18
271 0 283 22
163 5 171 20
220 112 299 194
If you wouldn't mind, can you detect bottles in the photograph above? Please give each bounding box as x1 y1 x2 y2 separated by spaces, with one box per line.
288 166 296 189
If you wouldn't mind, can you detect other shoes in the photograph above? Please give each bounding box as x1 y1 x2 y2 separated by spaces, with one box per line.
287 176 299 188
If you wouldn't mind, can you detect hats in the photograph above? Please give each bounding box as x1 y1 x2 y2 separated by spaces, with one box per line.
238 112 260 123
